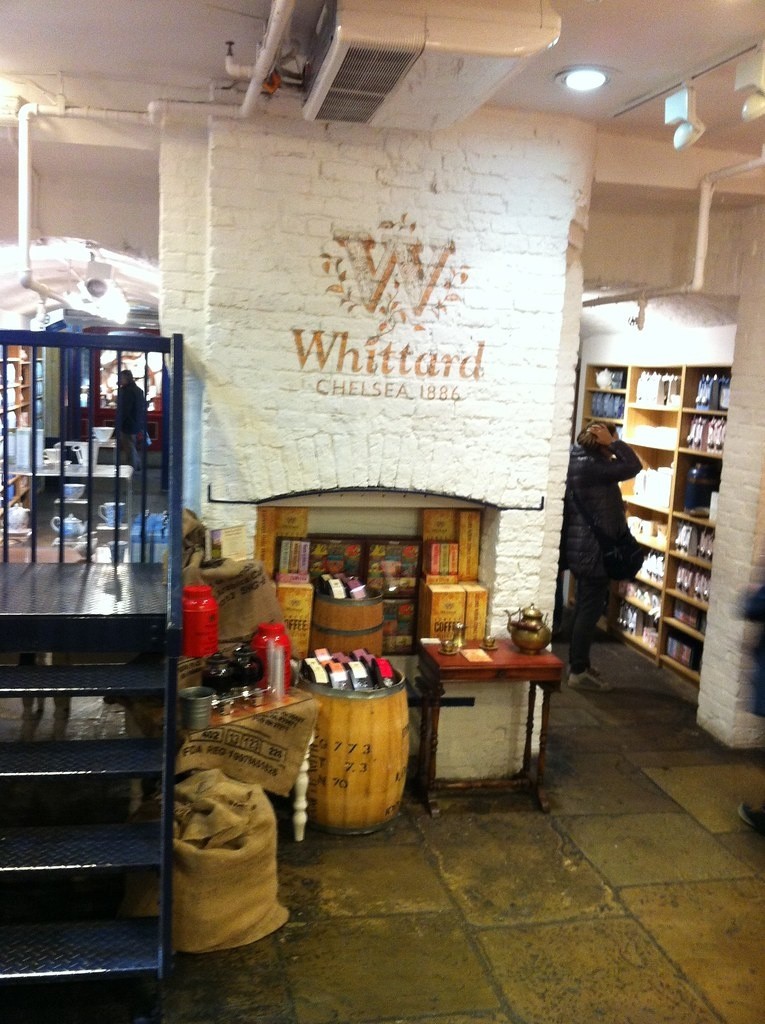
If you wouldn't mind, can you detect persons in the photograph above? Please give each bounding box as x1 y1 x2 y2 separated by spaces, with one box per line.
737 586 765 828
561 421 643 692
120 370 146 471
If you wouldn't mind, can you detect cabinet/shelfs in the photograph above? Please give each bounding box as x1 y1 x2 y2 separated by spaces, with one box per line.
578 362 733 686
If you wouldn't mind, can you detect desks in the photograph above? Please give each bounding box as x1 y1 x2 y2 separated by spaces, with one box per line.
409 639 563 817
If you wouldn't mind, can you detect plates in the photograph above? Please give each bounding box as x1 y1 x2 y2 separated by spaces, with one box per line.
1 529 30 537
478 642 499 650
437 648 458 656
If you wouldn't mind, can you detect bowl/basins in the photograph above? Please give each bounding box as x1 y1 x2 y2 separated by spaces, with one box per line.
92 427 115 442
64 483 86 501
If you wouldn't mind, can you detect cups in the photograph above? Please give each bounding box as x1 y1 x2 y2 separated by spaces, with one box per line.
265 638 285 698
109 541 128 563
441 640 454 651
43 449 60 460
484 635 496 647
98 502 125 527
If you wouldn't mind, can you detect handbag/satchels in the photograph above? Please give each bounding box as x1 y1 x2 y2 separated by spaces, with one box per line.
597 531 644 579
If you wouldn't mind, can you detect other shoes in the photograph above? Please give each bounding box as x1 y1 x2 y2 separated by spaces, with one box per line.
567 669 611 692
589 667 600 677
738 802 756 828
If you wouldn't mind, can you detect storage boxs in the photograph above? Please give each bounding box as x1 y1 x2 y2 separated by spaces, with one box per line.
256 506 314 661
417 508 486 643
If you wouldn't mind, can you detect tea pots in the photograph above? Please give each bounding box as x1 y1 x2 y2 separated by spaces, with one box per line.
49 513 88 541
6 503 31 531
502 603 553 655
595 368 616 390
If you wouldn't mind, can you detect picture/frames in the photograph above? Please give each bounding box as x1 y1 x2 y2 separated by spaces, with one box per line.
305 534 423 655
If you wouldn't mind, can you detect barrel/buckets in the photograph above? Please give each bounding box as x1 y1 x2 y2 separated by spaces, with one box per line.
308 579 384 661
181 585 218 657
250 623 291 696
296 668 410 836
178 686 215 731
